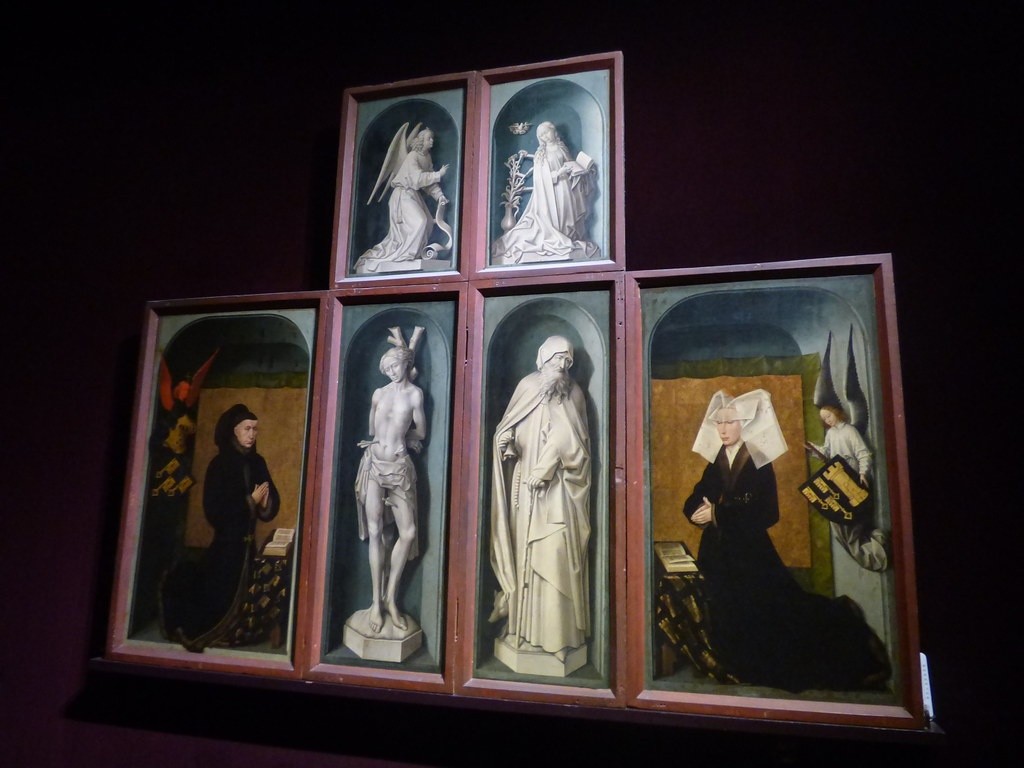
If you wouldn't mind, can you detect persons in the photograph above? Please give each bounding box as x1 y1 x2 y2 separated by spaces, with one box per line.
491 122 596 257
354 347 426 633
359 123 450 261
492 336 591 662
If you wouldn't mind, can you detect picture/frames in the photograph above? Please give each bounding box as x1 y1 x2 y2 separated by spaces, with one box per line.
476 50 627 280
104 290 326 681
327 71 475 290
304 282 464 694
624 251 928 730
461 272 626 711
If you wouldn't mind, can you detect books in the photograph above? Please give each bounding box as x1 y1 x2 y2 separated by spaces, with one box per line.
563 151 594 177
